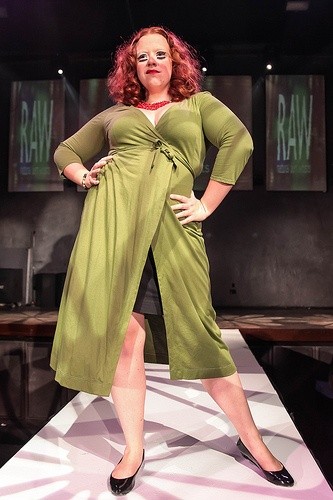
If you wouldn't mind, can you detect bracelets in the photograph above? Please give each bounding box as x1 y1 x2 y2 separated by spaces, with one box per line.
81 172 87 188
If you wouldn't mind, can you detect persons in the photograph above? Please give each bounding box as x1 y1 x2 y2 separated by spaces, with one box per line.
49 26 295 496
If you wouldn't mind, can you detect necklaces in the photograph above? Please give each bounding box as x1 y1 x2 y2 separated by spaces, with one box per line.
135 99 170 111
